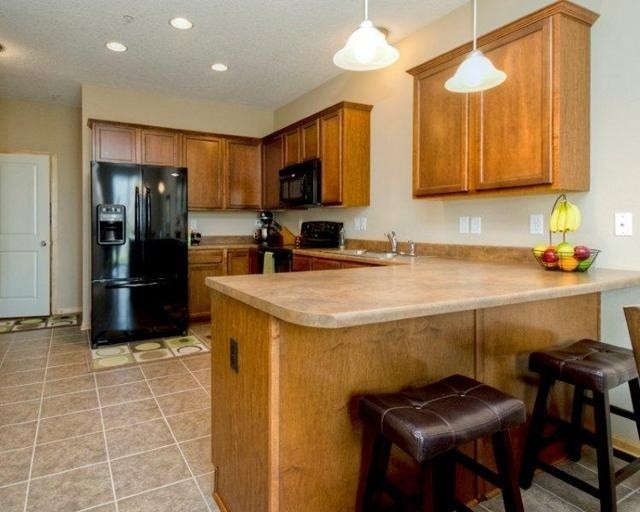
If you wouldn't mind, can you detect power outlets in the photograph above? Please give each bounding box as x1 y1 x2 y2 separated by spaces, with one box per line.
470 216 482 235
360 217 367 231
530 213 544 235
459 216 470 235
354 216 361 230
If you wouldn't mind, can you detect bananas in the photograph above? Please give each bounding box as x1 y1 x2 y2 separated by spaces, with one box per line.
550 199 580 233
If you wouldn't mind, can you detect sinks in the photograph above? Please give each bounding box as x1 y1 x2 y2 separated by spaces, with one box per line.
325 250 410 259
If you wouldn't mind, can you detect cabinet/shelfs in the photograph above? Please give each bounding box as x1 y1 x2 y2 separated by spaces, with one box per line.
92 124 184 165
262 125 301 209
291 256 341 271
189 250 252 319
300 109 343 205
411 14 592 202
182 134 264 212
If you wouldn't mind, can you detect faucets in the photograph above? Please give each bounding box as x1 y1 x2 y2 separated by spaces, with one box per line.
384 230 397 252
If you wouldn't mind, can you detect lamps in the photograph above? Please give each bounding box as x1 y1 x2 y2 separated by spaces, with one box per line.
333 1 401 73
444 0 508 94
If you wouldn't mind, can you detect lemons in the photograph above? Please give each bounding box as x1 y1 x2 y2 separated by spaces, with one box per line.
558 258 578 270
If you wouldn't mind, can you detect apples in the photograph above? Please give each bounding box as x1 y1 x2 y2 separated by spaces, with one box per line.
543 250 557 263
575 246 590 259
555 244 574 257
533 245 548 256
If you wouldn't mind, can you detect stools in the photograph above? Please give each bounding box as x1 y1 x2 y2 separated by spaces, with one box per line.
354 375 528 511
517 337 639 511
